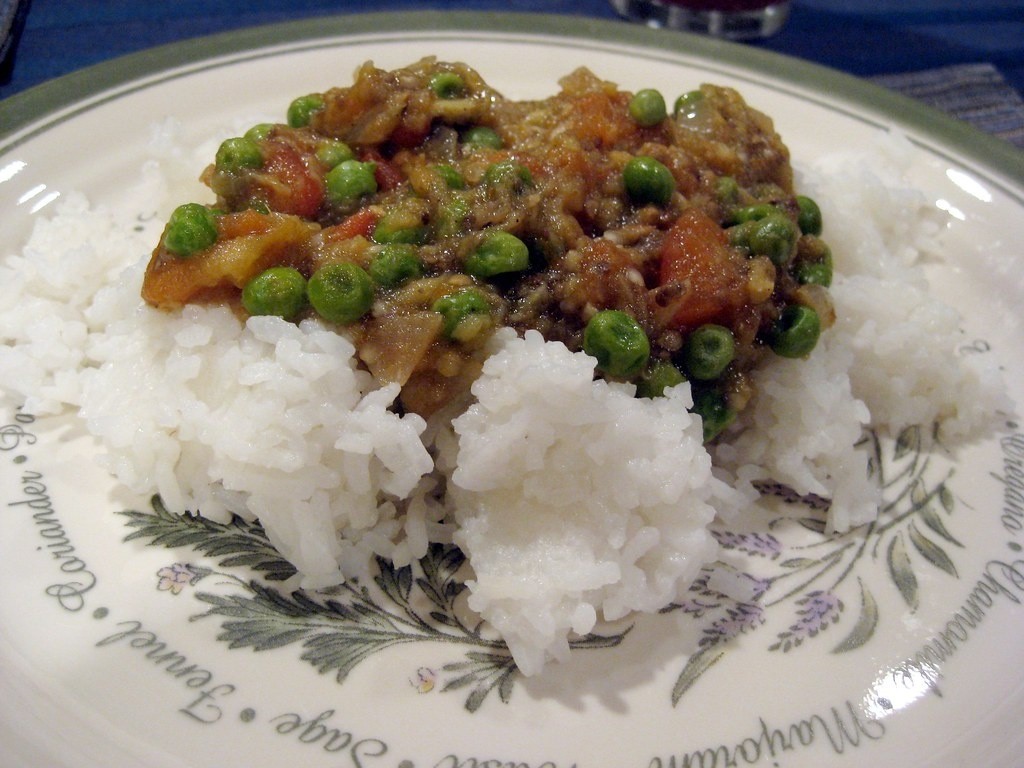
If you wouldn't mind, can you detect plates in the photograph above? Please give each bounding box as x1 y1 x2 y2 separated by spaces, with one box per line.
0 8 1024 767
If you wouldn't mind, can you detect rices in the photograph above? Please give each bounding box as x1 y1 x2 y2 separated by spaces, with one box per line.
0 55 1024 679
